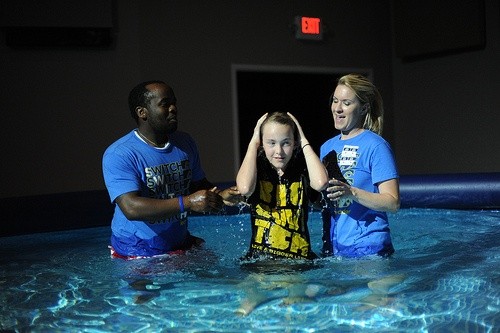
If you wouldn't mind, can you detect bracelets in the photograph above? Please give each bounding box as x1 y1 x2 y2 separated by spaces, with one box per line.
302 143 311 149
178 196 184 212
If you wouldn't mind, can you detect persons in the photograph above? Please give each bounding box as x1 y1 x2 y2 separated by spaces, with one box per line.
236 112 329 315
102 79 245 276
317 74 405 310
